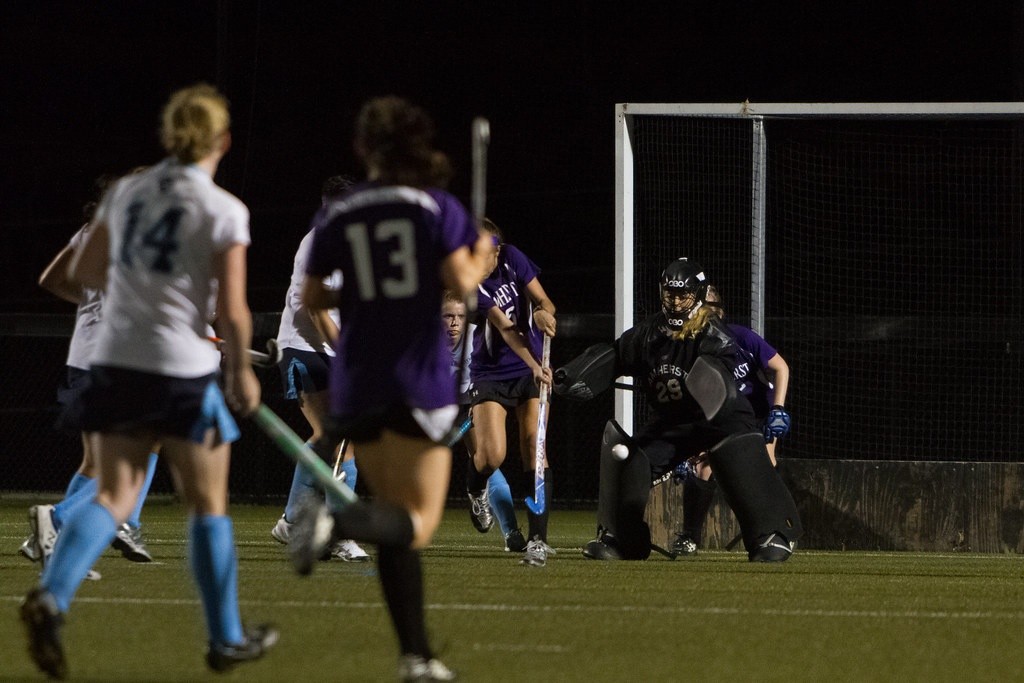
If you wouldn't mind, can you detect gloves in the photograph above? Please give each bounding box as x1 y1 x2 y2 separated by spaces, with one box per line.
764 405 790 443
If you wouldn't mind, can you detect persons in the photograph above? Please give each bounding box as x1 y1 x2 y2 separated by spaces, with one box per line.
669 285 789 552
439 289 528 554
20 86 282 682
19 165 160 582
460 219 556 568
285 99 495 682
271 173 370 565
553 260 806 561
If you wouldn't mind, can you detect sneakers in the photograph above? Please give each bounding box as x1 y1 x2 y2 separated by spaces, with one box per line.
503 530 527 551
272 512 295 544
466 478 496 534
331 537 373 564
28 503 60 564
399 655 454 681
17 589 63 673
109 521 153 562
519 538 556 566
19 532 43 562
290 487 336 577
207 624 280 671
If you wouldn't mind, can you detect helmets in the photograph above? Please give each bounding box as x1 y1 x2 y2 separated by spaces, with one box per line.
660 257 707 331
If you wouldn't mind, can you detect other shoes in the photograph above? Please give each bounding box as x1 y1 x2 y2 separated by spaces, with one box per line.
673 537 698 556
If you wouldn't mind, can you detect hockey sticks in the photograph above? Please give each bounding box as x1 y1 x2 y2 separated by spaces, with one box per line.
207 336 284 369
650 455 707 489
448 417 474 446
226 383 378 512
524 331 552 517
333 437 348 484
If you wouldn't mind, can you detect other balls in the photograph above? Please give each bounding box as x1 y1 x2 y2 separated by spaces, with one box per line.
611 444 629 461
